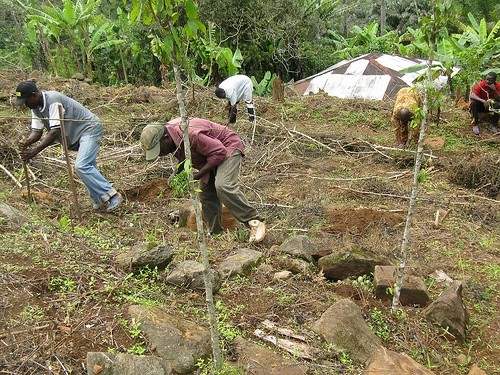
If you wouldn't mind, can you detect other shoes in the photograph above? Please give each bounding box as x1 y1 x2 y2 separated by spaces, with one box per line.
107 195 123 213
473 126 479 134
92 203 100 209
489 124 500 134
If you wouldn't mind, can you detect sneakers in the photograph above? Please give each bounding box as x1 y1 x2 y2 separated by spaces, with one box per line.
248 220 265 243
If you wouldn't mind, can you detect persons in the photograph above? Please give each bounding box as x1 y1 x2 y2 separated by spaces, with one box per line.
215 74 255 125
391 88 424 148
140 116 266 244
469 72 500 134
14 81 123 212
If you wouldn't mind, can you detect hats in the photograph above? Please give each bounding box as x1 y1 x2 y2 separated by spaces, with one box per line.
15 81 36 106
140 122 164 160
